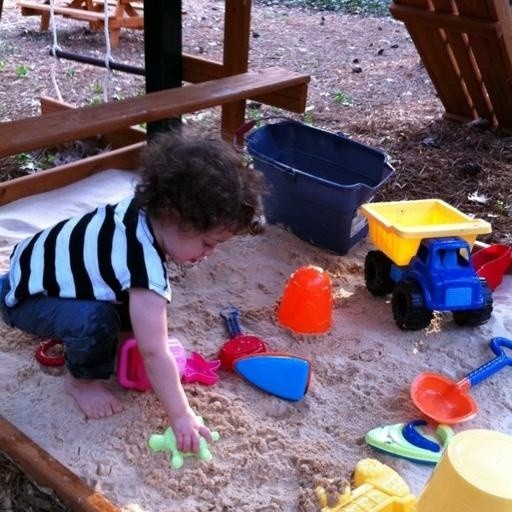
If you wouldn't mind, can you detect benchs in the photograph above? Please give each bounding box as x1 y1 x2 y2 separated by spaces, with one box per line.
16 1 105 34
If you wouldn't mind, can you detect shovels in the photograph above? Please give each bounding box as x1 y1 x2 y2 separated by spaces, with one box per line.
409 334 512 424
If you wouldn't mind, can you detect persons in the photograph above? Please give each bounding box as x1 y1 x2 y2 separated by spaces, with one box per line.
0 127 270 457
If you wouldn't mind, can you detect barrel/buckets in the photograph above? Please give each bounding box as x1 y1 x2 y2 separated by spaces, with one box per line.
412 428 512 512
